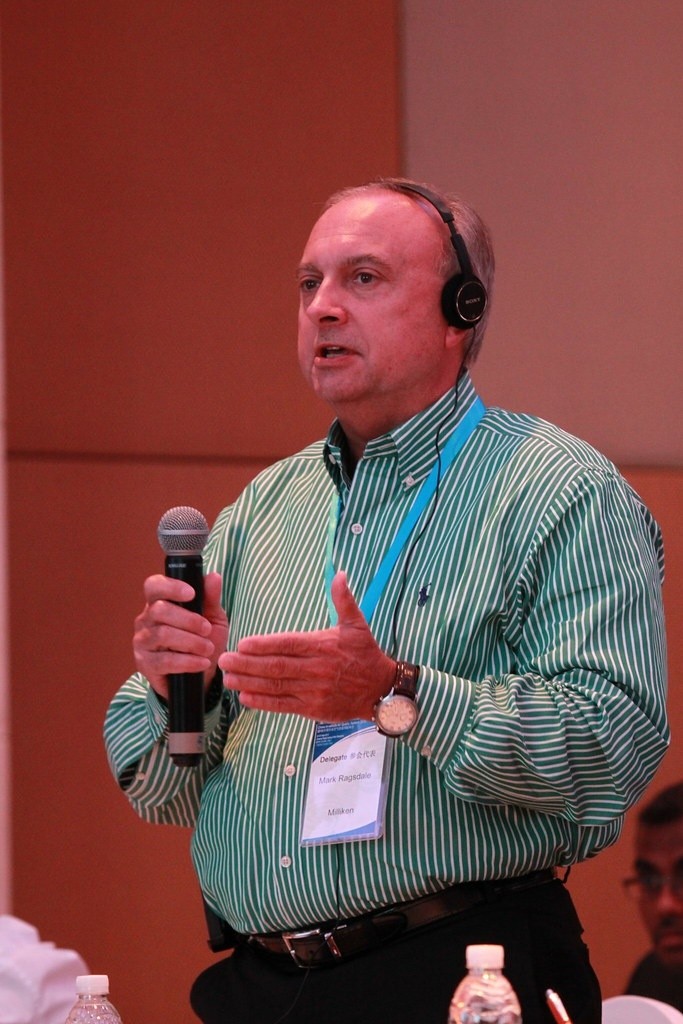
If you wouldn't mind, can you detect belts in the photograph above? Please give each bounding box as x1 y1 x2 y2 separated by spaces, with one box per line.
250 866 559 969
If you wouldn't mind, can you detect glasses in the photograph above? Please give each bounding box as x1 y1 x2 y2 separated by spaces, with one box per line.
622 857 683 904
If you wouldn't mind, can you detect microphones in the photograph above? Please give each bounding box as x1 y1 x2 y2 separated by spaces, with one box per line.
157 506 210 769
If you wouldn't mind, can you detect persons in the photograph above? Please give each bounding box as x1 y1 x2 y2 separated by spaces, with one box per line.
615 784 683 1013
98 175 671 1024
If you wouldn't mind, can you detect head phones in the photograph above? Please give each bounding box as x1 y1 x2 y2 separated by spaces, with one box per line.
378 180 487 332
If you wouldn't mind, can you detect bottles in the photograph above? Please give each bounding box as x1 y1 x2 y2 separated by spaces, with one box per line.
66 975 122 1024
447 947 521 1024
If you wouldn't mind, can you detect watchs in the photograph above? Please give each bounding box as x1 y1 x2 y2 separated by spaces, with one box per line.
370 662 421 738
